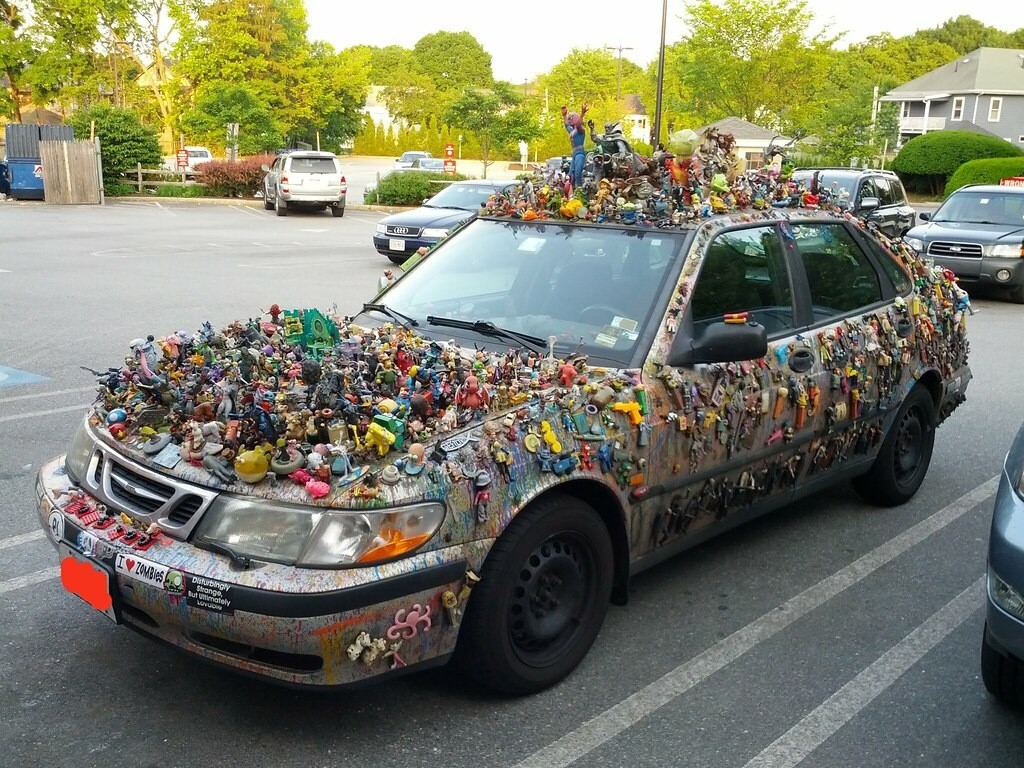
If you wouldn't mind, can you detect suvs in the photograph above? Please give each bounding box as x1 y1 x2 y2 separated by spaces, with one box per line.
262 151 348 217
789 167 918 240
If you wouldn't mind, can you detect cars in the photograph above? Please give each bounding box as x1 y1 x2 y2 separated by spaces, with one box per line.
978 420 1024 707
372 180 537 265
32 191 976 700
542 156 573 179
900 183 1024 303
395 150 434 170
404 158 444 173
183 146 212 172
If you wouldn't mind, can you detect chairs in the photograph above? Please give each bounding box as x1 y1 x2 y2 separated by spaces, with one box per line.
691 247 764 325
797 251 874 314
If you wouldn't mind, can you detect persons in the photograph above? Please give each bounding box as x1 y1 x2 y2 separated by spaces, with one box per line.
96 102 980 549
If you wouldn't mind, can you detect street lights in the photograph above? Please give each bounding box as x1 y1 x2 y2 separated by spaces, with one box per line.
607 44 634 102
101 41 130 109
522 78 529 115
